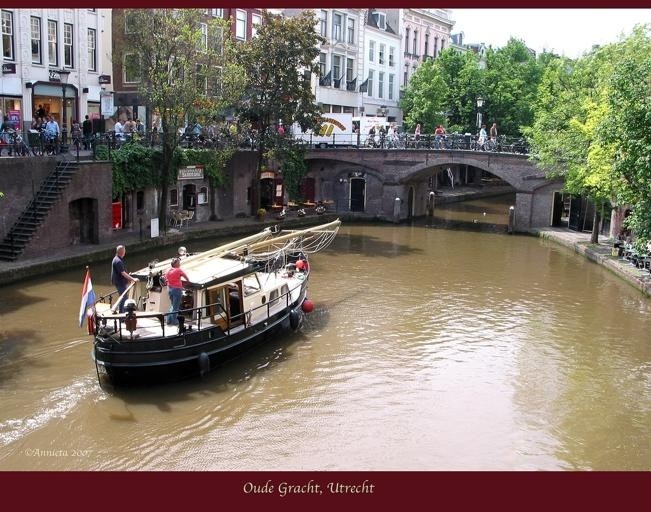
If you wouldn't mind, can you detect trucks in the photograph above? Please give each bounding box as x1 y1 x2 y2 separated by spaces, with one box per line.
278 111 353 149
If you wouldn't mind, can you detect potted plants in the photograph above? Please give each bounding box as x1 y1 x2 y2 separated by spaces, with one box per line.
258 209 266 222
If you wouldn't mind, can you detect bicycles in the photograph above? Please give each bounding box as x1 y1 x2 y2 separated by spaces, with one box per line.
2 127 122 160
364 131 532 153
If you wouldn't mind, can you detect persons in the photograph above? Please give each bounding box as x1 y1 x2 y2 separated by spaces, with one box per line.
0 103 285 156
111 244 139 314
166 258 192 326
364 120 498 153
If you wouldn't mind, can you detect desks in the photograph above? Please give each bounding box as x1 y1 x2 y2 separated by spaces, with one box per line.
272 200 336 217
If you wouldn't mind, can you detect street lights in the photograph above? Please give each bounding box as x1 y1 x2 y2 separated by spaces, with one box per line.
476 94 484 135
58 66 71 154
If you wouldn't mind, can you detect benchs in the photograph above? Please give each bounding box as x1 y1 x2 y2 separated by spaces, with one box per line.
0 144 23 156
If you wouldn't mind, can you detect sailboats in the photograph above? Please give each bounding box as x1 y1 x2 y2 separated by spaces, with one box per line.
76 214 344 392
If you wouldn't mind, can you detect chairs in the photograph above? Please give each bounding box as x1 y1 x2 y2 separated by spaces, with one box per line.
169 209 194 231
